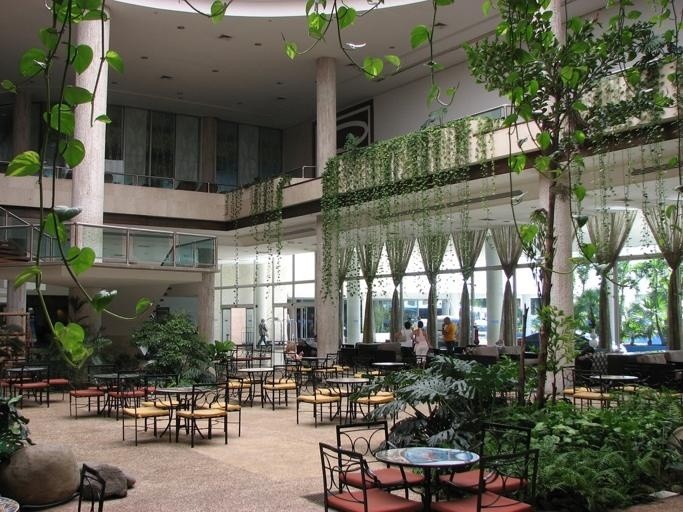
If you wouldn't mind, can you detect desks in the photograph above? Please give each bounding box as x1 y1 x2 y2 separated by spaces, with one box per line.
0 496 20 512
376 447 481 512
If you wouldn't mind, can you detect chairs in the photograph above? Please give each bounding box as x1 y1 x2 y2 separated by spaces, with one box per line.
78 463 106 512
319 442 424 512
431 449 539 512
0 359 243 448
437 422 531 501
336 421 426 499
561 366 638 413
215 344 428 428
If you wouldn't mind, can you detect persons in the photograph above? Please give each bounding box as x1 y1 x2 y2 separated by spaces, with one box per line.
256 319 269 348
286 338 308 361
394 317 433 370
441 316 463 356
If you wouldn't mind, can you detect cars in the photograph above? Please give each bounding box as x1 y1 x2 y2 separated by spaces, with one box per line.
518 333 624 351
417 313 535 344
